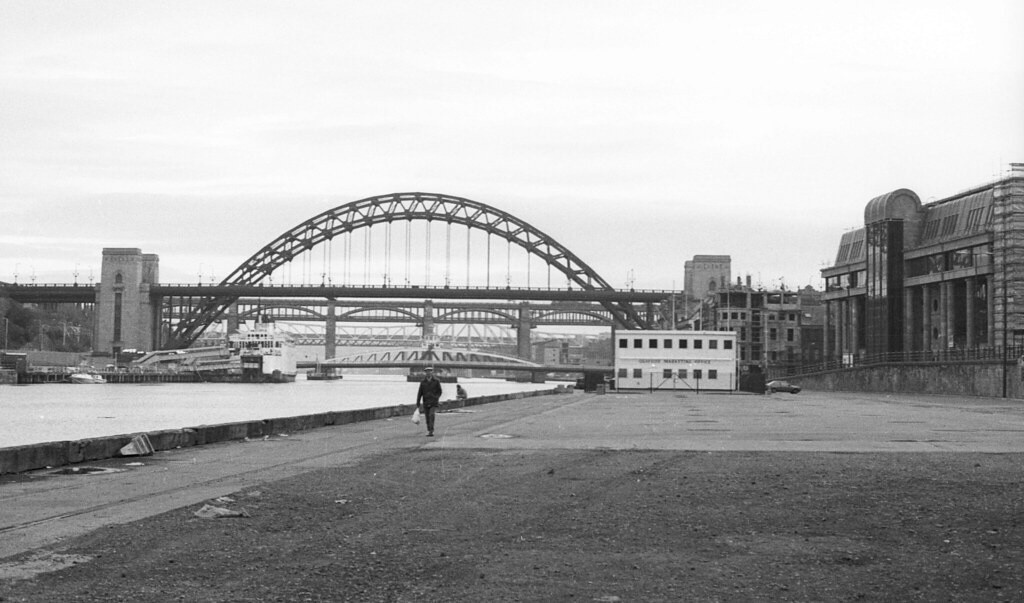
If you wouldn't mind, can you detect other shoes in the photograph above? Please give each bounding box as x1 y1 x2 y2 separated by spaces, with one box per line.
429 429 434 436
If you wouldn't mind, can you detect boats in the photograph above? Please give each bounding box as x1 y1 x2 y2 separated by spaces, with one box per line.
304 361 344 380
405 347 459 384
70 367 110 385
119 287 298 384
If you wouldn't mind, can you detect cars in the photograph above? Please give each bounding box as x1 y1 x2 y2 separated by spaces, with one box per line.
765 380 802 394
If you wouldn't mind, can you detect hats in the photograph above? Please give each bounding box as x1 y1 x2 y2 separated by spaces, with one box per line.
424 367 433 370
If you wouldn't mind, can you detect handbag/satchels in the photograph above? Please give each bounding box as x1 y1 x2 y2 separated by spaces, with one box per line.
412 406 420 424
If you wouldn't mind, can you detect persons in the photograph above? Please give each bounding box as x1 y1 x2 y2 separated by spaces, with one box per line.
456 384 467 399
415 366 442 437
